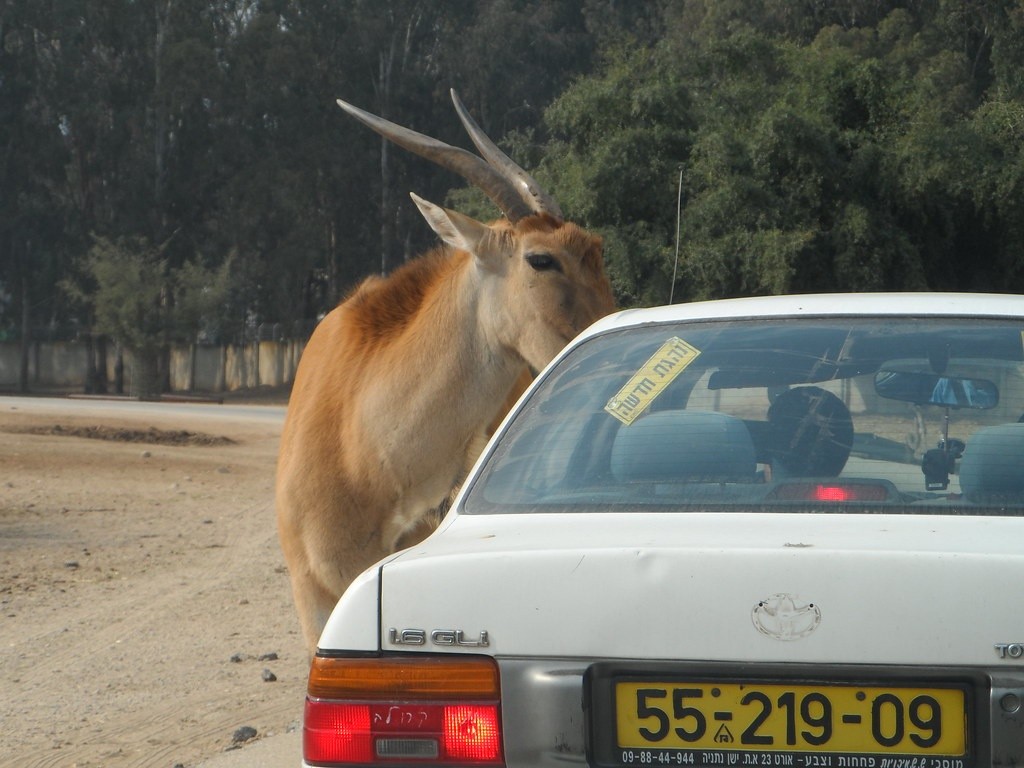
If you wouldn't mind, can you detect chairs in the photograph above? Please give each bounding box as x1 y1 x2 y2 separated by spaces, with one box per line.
960 422 1024 500
613 409 761 486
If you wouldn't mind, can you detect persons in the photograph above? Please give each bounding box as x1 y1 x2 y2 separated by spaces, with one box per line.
757 387 855 479
932 378 999 407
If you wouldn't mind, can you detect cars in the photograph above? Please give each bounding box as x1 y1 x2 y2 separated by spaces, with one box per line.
296 289 1024 768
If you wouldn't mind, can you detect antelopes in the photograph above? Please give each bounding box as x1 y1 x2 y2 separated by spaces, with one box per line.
276 85 614 664
